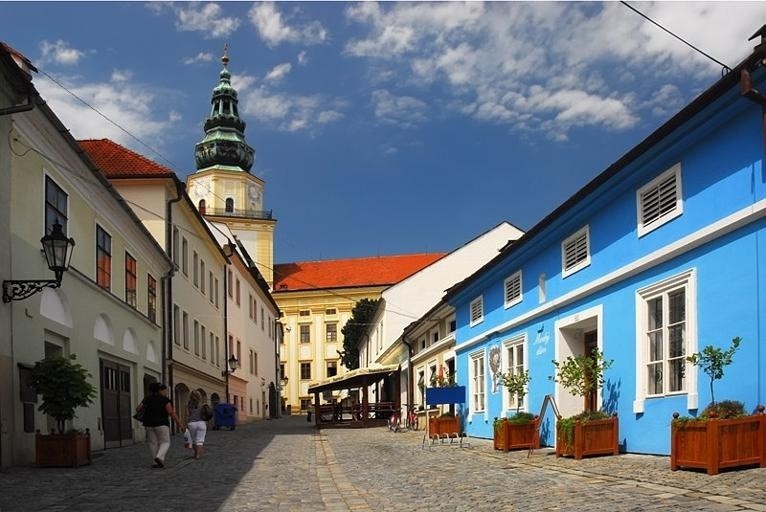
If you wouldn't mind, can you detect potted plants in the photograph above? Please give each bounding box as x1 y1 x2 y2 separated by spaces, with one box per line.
670 336 766 475
494 370 539 452
429 370 461 440
26 354 97 468
547 345 619 460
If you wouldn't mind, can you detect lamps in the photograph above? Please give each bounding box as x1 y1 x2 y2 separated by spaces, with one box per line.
222 354 240 377
2 219 76 305
279 376 288 387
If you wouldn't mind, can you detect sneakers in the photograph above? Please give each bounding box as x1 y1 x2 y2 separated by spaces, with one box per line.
152 464 160 469
154 458 164 468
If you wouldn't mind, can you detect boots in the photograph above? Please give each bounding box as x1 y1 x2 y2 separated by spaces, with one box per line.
193 444 203 459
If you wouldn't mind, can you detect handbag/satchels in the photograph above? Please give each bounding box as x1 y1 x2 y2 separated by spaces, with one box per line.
201 404 213 422
133 404 148 421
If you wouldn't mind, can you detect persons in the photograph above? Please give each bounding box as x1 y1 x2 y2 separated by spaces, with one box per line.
184 388 208 460
135 382 186 468
305 399 314 425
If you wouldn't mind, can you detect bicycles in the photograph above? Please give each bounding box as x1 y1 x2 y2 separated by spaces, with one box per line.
402 403 420 430
386 405 403 432
